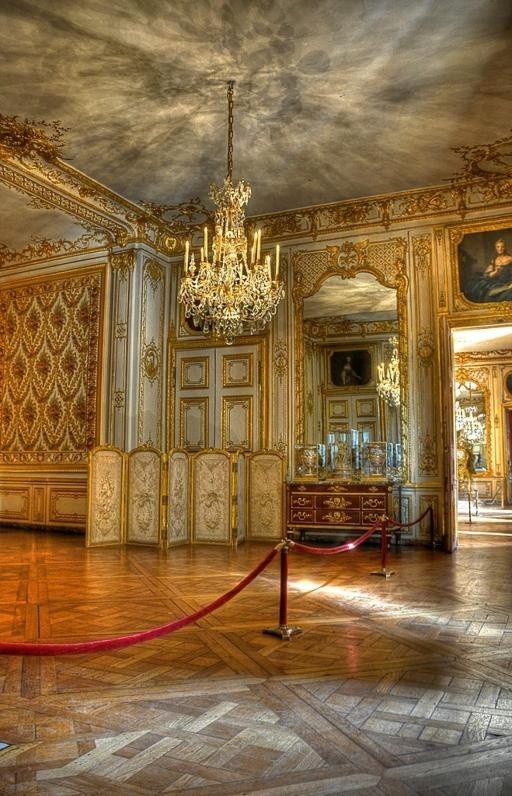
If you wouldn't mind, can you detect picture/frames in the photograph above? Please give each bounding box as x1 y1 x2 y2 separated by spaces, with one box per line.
447 215 511 313
322 343 382 394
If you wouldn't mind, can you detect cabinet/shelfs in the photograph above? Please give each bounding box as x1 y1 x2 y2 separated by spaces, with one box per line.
283 478 401 579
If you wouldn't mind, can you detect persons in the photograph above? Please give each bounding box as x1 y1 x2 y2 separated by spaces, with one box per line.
335 355 363 386
471 238 512 302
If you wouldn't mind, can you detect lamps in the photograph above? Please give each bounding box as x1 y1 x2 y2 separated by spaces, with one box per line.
177 83 284 349
